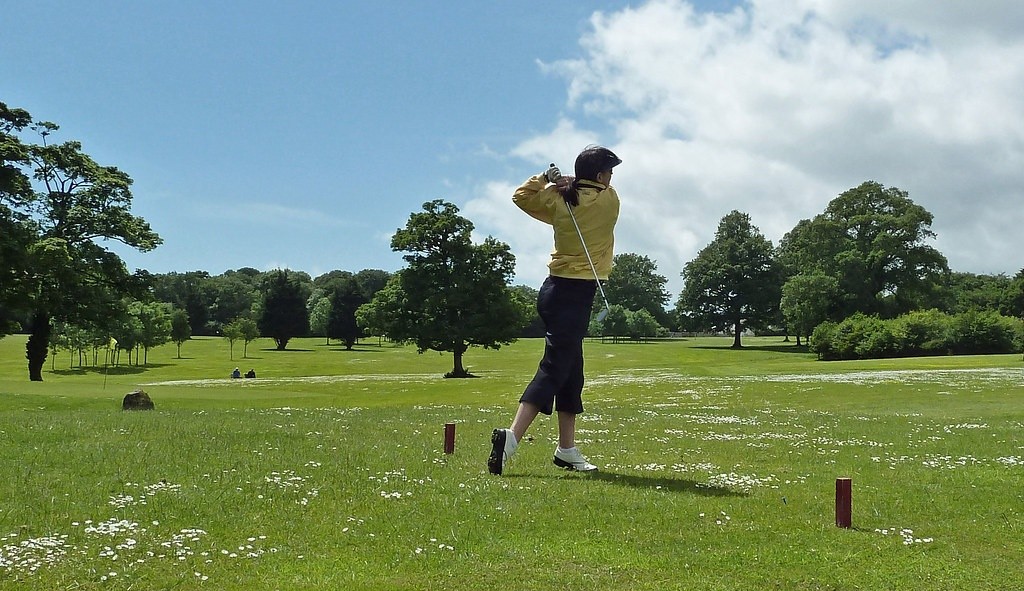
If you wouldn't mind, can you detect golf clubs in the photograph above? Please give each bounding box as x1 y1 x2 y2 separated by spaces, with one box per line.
550 162 613 322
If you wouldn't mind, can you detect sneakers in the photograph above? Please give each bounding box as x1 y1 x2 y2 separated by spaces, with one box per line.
487 429 518 476
553 445 598 474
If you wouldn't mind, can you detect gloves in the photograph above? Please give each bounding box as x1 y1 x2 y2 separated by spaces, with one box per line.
543 167 575 191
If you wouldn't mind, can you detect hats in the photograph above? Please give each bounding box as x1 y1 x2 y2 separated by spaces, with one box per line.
575 147 622 174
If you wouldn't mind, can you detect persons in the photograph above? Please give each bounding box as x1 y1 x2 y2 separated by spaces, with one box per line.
245 369 256 378
488 146 624 476
232 367 240 378
